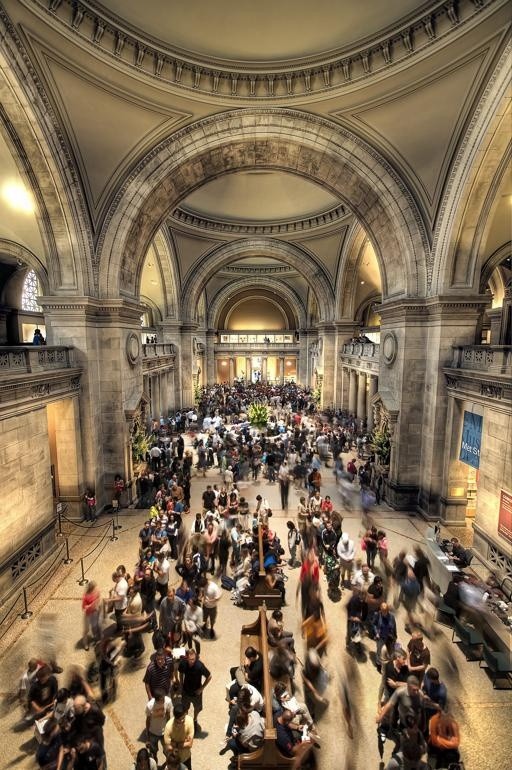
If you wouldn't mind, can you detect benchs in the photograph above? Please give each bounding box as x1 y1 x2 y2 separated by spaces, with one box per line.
236 512 301 769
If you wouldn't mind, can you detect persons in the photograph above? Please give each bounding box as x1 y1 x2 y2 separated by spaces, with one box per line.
154 334 157 343
350 336 372 344
151 337 154 344
14 375 474 769
32 328 45 347
146 336 149 343
263 337 267 343
267 337 270 343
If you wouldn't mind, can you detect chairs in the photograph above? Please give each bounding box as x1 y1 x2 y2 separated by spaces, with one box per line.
434 593 512 691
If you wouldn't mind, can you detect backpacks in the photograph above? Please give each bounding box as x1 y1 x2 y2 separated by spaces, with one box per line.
291 529 300 544
221 575 234 589
